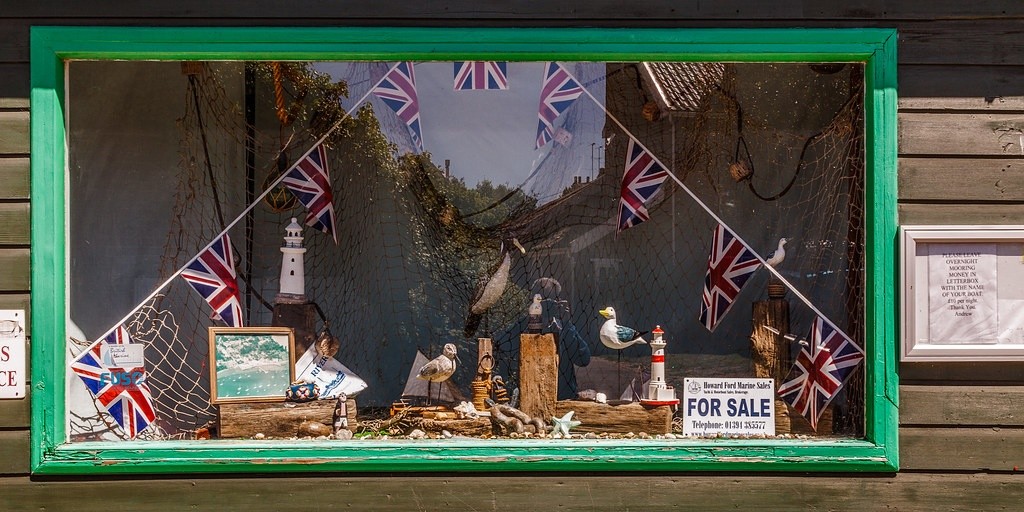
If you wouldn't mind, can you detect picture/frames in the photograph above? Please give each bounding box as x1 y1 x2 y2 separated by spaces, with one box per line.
209 327 297 404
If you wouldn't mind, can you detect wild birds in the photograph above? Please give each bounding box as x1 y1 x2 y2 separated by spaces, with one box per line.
598 306 650 350
416 343 462 382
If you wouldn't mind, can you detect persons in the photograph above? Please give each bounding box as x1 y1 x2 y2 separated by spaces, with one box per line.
492 278 590 403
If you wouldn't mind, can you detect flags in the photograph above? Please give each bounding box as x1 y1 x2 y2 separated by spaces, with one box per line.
698 224 761 335
778 316 864 432
372 61 423 152
616 137 670 234
454 60 508 89
70 327 155 439
533 62 584 150
180 233 243 327
281 142 340 245
